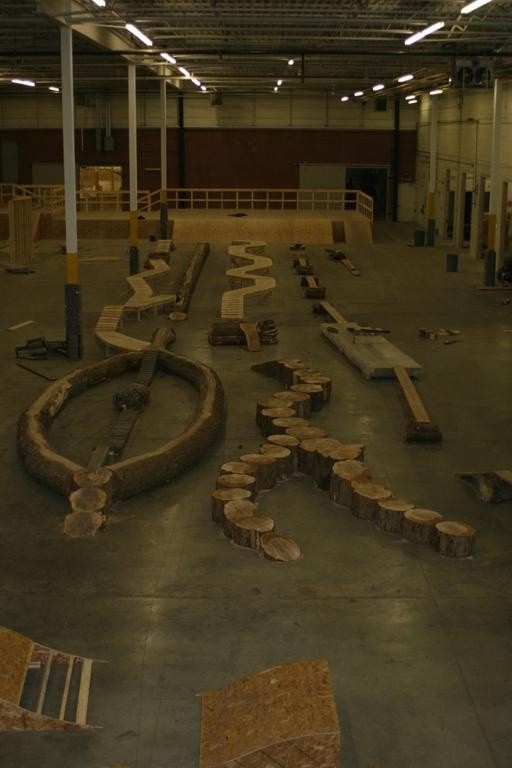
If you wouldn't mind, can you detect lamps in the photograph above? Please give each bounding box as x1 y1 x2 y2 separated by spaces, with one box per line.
10 0 207 94
273 0 491 106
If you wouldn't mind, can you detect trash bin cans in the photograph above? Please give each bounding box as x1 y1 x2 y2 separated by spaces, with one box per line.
415 230 425 246
447 254 458 272
